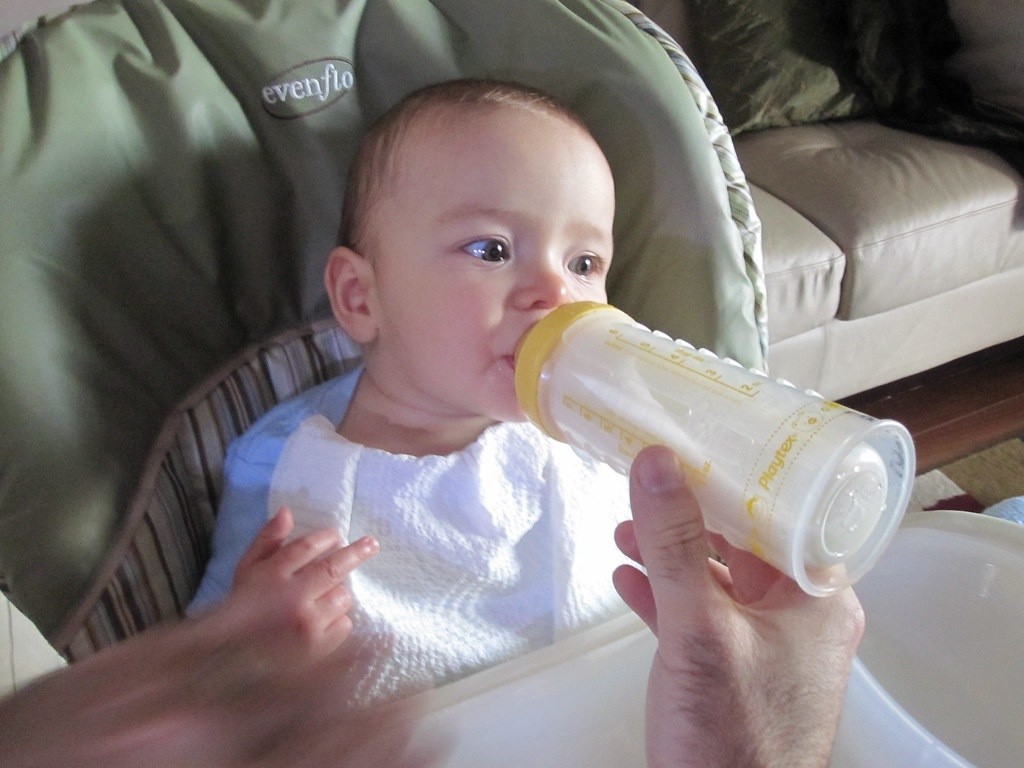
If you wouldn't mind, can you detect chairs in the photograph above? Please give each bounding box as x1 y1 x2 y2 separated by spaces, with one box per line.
0 0 773 643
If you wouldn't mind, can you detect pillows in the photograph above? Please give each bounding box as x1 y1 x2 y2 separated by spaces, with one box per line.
687 0 872 135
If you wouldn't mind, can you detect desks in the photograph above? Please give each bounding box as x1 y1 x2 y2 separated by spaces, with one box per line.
252 508 1024 768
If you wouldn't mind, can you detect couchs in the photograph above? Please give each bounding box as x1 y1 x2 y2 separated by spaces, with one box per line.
621 0 1024 404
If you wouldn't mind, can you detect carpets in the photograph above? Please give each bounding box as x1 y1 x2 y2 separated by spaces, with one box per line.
903 432 1024 525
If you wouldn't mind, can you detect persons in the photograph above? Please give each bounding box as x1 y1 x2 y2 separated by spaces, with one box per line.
183 77 711 718
0 445 865 768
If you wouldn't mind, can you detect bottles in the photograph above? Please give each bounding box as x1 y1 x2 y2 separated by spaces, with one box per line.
512 302 919 598
